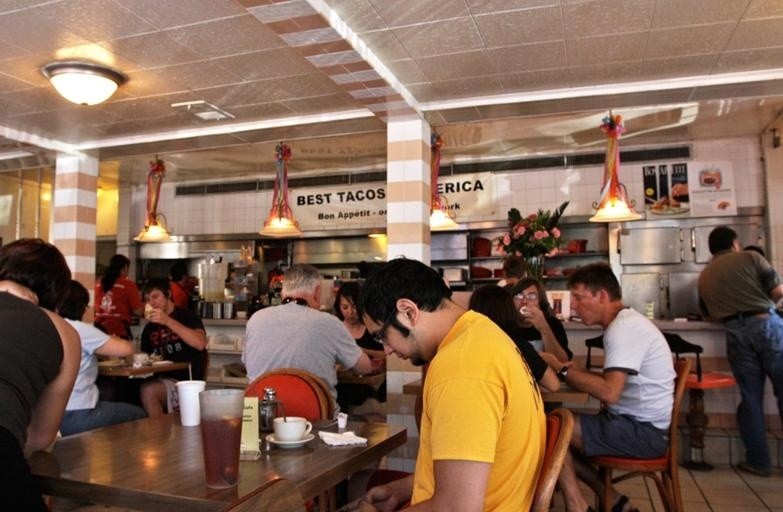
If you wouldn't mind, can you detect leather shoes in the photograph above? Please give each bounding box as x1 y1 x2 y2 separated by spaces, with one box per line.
738 462 769 477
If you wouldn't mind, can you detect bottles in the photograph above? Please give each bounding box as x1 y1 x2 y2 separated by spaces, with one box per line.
257 385 288 435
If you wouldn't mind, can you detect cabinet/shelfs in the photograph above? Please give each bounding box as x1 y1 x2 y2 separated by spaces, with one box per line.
470 251 609 283
201 318 250 391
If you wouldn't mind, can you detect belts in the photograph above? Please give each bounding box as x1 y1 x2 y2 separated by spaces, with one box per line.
722 309 769 324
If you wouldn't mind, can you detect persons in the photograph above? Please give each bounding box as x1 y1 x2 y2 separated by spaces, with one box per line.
0 238 81 512
56 279 146 437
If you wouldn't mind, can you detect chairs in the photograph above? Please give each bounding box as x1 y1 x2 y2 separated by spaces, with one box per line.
562 332 736 470
586 356 691 511
533 407 574 512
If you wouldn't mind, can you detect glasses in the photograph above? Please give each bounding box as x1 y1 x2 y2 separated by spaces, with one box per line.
281 296 310 307
373 308 394 345
513 293 537 300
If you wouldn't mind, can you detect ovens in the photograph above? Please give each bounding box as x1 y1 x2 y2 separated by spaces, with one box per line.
607 217 765 318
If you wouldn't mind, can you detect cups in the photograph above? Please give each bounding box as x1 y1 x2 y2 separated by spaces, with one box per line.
553 299 563 315
337 412 349 429
175 379 208 428
199 390 245 491
271 414 313 442
644 299 656 320
131 353 149 364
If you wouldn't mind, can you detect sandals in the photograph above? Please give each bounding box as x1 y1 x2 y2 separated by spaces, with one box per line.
611 495 639 512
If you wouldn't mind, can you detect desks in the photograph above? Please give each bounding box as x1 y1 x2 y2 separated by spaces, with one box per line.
25 410 408 512
90 327 601 425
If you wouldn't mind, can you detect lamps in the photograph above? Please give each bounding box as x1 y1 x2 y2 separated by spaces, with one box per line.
256 134 303 242
585 113 646 226
428 130 458 234
130 154 175 246
38 59 129 111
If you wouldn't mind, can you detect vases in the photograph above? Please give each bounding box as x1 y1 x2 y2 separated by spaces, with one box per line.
529 252 547 282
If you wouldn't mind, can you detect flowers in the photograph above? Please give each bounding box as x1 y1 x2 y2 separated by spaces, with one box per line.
488 201 574 261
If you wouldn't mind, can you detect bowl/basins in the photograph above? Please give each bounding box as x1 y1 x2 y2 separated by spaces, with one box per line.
471 267 493 277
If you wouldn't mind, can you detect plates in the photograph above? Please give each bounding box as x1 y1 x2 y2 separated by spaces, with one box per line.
265 431 314 451
648 201 691 216
